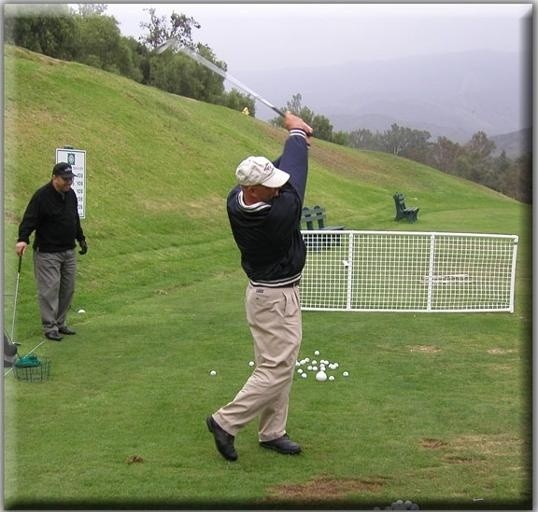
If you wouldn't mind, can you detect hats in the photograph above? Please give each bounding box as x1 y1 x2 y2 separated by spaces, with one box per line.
52 162 79 177
235 156 291 190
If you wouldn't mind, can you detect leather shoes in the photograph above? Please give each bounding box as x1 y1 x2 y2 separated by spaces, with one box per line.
206 415 238 461
260 434 301 454
45 331 63 341
59 326 75 335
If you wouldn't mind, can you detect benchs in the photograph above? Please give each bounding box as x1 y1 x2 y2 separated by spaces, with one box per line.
393 193 419 223
299 205 345 251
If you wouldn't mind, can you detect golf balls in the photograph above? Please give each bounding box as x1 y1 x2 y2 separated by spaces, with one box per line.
249 361 255 366
296 350 349 381
210 369 217 376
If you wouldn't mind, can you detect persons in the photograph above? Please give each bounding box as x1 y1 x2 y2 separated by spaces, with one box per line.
205 107 316 463
13 162 87 340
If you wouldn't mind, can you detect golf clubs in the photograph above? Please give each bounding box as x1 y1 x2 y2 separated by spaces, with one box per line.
155 38 313 138
69 306 87 314
10 249 23 347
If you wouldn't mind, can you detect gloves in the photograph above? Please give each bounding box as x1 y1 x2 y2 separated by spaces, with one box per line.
78 240 87 255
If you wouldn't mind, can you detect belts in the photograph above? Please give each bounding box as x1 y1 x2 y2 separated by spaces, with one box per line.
272 279 300 288
